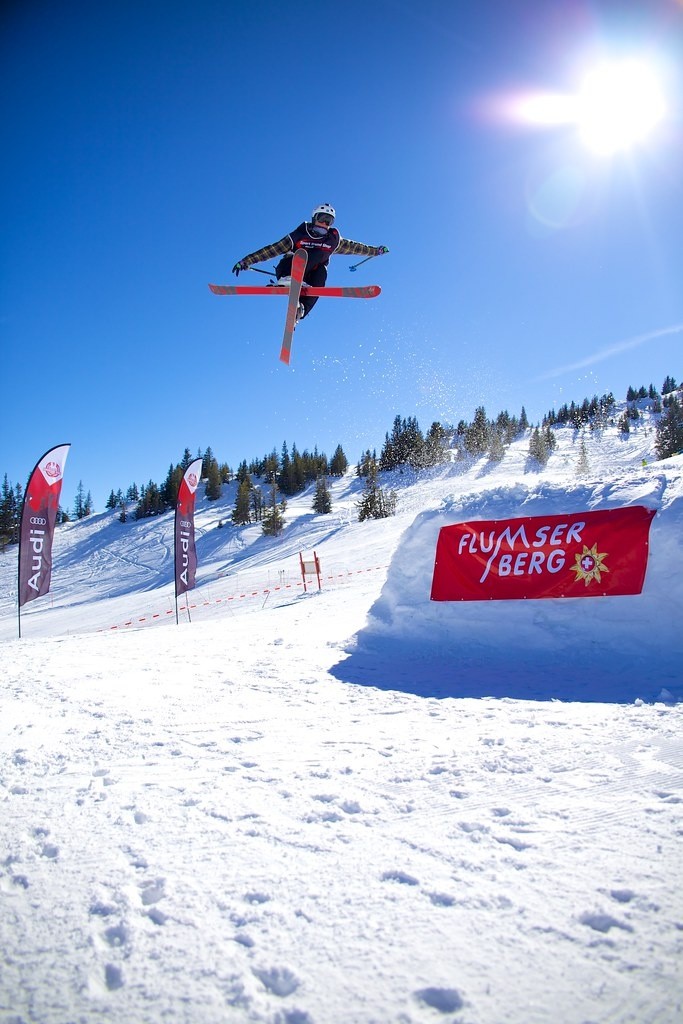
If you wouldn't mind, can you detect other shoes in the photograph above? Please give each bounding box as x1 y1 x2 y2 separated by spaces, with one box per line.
278 276 291 287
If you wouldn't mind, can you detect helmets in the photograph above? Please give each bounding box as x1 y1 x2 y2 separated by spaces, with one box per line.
311 203 335 218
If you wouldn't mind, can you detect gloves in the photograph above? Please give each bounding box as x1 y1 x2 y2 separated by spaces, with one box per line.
231 260 245 277
378 246 389 255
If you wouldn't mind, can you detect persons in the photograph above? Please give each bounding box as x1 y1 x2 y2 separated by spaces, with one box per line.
232 205 384 320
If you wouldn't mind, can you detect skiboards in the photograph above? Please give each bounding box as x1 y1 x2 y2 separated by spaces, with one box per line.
207 248 382 367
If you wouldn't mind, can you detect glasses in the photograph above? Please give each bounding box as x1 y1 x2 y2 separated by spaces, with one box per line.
314 214 334 226
